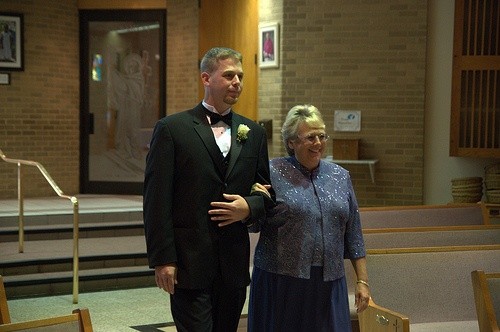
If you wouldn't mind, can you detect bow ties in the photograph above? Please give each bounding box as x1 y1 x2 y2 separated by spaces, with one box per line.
201 103 233 126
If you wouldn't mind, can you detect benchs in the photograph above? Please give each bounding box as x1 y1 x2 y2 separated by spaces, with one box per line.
344 203 500 332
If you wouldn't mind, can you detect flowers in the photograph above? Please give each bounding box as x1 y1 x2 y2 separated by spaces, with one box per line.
236 124 251 143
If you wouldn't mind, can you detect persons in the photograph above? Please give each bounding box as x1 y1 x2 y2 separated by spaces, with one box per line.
142 48 276 332
247 104 369 332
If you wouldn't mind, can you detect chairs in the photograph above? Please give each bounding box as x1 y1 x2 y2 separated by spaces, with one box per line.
0 308 93 332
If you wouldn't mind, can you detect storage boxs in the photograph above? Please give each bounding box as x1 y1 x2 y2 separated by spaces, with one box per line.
332 138 361 160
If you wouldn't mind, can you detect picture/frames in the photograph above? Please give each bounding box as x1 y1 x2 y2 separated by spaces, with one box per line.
257 23 279 69
0 11 24 71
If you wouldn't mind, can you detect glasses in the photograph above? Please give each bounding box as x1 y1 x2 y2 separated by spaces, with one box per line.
296 134 330 143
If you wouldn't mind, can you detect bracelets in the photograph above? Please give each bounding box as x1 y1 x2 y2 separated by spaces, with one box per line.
356 280 368 286
